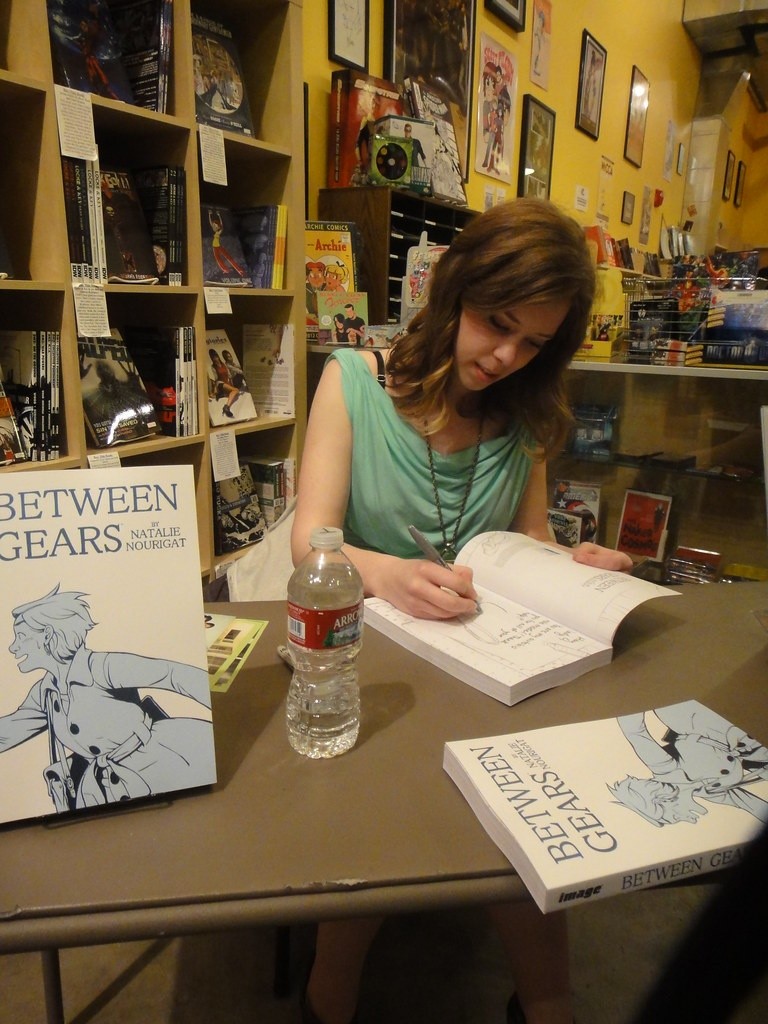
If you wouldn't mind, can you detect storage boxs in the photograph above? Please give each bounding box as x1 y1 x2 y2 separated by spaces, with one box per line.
574 338 621 358
628 289 768 367
327 66 435 197
565 403 620 457
554 477 603 544
547 507 590 548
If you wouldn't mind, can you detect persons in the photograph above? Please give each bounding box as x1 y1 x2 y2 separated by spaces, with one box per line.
291 197 633 1024
208 349 248 417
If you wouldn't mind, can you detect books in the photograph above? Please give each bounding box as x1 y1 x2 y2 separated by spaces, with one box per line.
0 324 200 471
303 68 469 351
442 698 768 914
46 0 286 290
205 329 257 427
364 531 683 708
212 454 295 556
242 324 296 419
0 464 219 827
546 479 604 548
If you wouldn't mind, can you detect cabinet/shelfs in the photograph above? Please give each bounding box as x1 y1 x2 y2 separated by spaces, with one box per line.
317 184 484 326
0 0 307 583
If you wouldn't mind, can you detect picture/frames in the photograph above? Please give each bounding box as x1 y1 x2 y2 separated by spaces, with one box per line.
573 28 608 142
722 149 736 201
382 0 477 184
484 0 527 33
732 159 746 208
620 191 635 225
623 64 651 169
327 0 370 74
517 93 556 201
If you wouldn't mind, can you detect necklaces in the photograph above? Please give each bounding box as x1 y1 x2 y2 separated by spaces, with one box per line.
423 422 482 565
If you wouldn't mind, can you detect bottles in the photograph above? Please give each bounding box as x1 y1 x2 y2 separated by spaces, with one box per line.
286 527 364 759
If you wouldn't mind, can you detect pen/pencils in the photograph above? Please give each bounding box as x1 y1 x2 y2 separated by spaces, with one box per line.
407 525 482 613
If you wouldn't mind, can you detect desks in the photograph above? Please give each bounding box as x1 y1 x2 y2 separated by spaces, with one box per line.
560 361 768 490
0 578 768 1024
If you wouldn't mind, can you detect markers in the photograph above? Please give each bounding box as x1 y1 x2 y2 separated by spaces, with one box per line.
277 645 293 666
